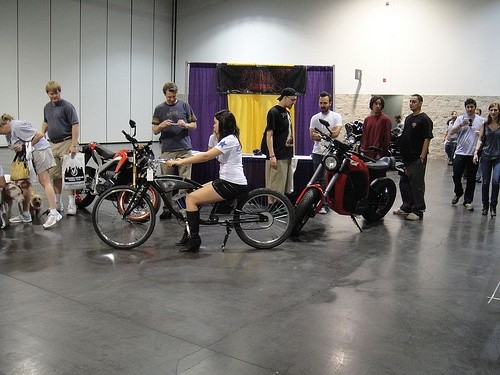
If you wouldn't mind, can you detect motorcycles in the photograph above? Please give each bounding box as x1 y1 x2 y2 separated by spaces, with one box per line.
346 119 405 171
69 119 161 224
288 117 405 238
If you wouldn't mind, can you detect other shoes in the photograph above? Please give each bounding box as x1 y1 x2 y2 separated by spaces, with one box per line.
66 204 77 214
48 202 63 211
393 208 410 214
160 212 173 219
9 214 32 223
465 203 474 211
319 203 330 213
451 191 464 204
482 203 489 215
405 213 421 220
490 202 497 216
475 178 481 182
42 211 62 228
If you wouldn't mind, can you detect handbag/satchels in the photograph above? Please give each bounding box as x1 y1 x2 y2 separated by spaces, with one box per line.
10 140 38 184
62 153 86 190
478 121 487 154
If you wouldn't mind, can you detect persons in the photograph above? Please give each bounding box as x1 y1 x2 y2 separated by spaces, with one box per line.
308 91 342 214
472 103 500 216
462 108 483 183
394 115 404 130
360 95 392 185
261 87 298 219
166 109 248 253
208 131 219 151
0 113 62 228
151 82 197 221
450 98 485 211
393 94 434 221
40 81 80 216
443 111 459 166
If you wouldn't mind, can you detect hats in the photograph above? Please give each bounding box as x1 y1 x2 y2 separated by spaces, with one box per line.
277 88 298 100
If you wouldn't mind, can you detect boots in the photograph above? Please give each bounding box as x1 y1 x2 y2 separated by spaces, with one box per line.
174 210 201 253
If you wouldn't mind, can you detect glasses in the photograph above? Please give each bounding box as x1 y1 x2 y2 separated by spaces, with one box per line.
286 96 298 102
490 109 498 111
468 119 472 127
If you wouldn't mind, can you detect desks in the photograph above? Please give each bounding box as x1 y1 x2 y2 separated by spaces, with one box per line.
191 154 313 207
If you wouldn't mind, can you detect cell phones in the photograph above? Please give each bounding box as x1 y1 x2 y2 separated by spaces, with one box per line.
171 123 177 126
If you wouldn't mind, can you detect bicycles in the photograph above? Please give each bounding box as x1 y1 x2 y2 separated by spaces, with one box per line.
91 160 295 253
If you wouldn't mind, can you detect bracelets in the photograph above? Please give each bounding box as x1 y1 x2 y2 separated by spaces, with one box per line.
270 154 275 158
71 144 76 147
185 124 188 129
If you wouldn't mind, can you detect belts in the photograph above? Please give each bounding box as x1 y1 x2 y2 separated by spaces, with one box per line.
46 136 72 144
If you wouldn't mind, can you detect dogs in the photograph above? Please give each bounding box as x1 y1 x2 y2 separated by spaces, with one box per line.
0 182 25 229
29 190 42 219
